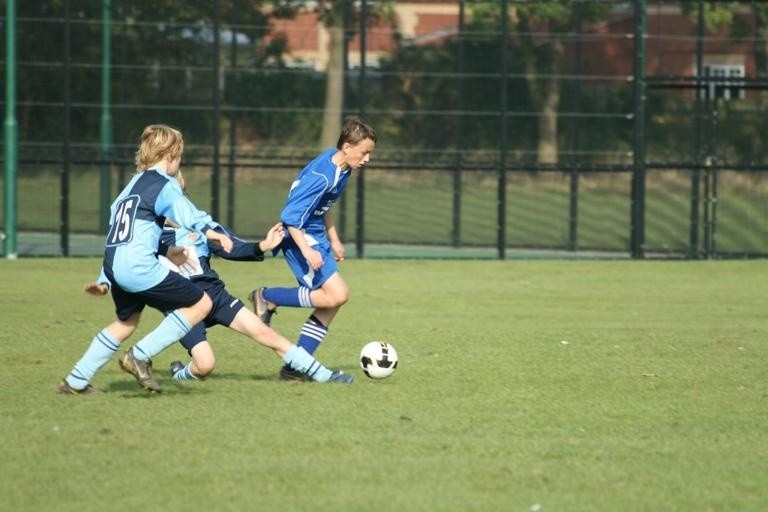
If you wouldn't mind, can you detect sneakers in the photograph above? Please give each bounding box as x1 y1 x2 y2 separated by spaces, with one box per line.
117 347 164 395
246 286 279 329
276 364 314 383
169 358 187 380
58 378 108 399
322 369 354 386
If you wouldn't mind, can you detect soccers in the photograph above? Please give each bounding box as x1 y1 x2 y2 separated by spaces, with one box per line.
359 342 398 379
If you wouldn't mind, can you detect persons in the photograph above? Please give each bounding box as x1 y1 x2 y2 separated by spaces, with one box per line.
249 120 379 380
82 166 355 384
56 123 235 398
